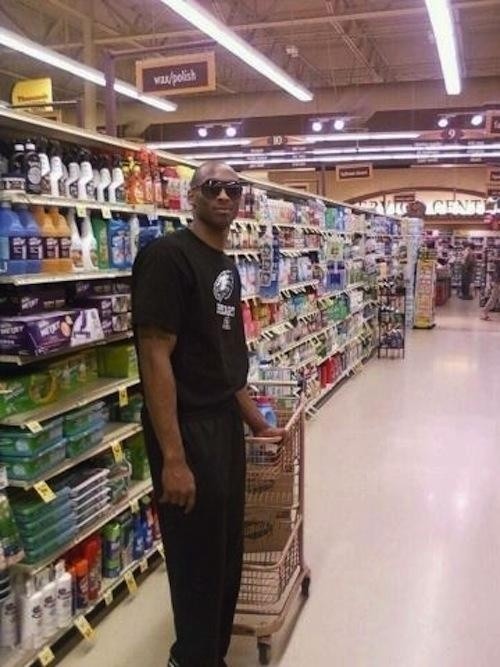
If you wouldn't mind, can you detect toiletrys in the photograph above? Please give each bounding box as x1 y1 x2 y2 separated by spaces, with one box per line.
23 134 42 193
0 560 73 650
10 135 25 175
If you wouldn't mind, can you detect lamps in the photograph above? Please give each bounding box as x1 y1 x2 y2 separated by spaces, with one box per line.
0 27 178 113
424 0 462 97
161 0 314 103
194 122 244 138
435 111 486 128
308 115 359 131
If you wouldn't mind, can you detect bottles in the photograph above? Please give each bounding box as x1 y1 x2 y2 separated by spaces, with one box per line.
25 143 41 194
10 143 24 176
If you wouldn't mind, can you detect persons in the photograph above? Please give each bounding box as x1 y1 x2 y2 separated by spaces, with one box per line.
460 243 477 300
479 260 500 321
129 160 286 667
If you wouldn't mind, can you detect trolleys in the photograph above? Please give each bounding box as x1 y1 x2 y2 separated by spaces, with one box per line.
374 278 406 359
211 376 312 662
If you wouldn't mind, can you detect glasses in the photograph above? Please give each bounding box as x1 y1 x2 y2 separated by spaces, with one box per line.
191 178 242 201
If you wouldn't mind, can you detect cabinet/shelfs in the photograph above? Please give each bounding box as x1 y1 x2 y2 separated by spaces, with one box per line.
0 103 406 667
426 236 500 313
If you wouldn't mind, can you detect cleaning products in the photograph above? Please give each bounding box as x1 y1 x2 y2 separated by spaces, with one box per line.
31 204 59 273
16 203 43 273
48 205 70 273
39 137 125 202
250 397 277 462
81 209 99 272
66 208 85 272
0 201 27 275
